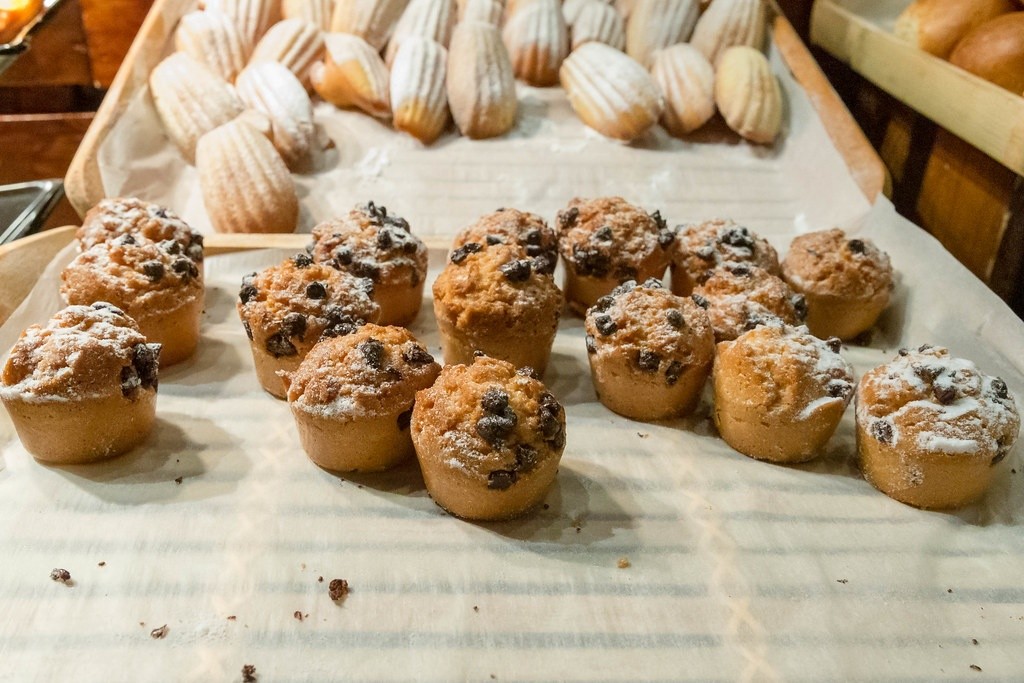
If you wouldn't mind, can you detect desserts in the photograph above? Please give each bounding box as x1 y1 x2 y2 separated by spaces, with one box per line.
0 196 1022 521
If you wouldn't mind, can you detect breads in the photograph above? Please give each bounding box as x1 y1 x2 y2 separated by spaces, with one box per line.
891 0 1024 102
0 0 159 92
149 0 783 228
